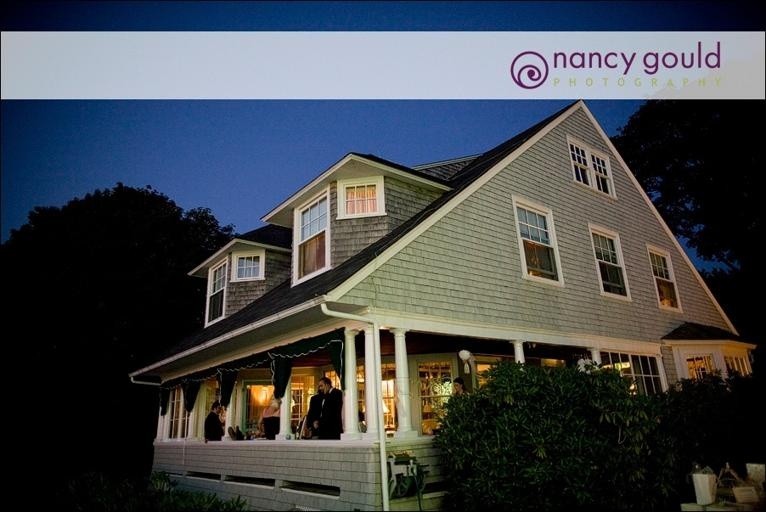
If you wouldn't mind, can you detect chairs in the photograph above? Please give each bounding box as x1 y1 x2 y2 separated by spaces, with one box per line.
226 414 309 441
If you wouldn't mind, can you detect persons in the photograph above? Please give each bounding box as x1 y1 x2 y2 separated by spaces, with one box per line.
205 400 225 441
453 377 470 395
228 419 265 440
310 376 343 439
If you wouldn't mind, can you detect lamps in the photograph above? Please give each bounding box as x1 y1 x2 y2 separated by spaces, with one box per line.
423 400 432 413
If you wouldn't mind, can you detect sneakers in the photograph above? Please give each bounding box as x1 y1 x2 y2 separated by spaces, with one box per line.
235 425 245 440
228 426 236 440
